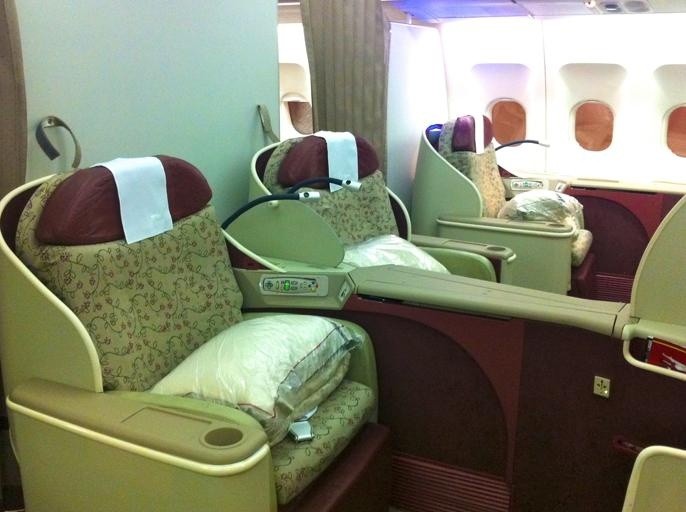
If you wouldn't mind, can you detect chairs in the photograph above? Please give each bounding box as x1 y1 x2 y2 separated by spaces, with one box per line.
0 155 404 512
414 115 594 300
247 130 518 287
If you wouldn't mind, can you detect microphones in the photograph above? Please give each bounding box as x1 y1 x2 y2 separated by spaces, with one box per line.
494 137 552 153
287 175 364 202
222 190 322 230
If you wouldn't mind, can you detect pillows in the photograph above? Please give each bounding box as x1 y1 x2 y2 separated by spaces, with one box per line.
143 314 359 443
